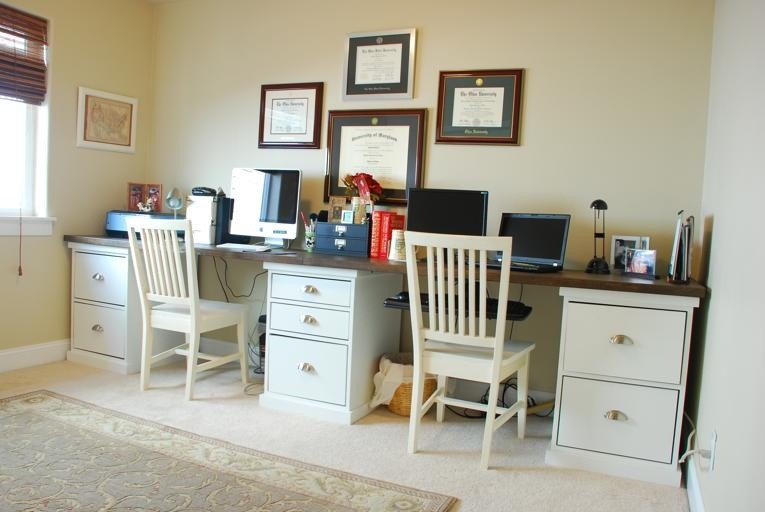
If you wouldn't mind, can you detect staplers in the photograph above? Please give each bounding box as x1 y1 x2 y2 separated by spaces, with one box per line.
192 187 216 196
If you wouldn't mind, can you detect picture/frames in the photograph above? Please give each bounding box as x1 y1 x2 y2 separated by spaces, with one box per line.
77 86 140 156
127 180 144 212
339 29 419 102
611 235 651 268
257 81 324 150
144 184 163 213
433 67 526 147
321 108 428 208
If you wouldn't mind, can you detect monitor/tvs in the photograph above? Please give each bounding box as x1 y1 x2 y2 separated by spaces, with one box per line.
406 187 488 264
230 167 302 249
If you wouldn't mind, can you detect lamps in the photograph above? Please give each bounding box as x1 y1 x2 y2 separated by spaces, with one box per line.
165 187 183 215
585 198 613 275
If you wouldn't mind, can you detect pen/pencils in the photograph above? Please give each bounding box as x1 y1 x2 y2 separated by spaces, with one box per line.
301 211 317 232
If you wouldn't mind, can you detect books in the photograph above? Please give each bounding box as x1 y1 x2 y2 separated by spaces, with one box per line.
370 211 392 258
380 214 405 259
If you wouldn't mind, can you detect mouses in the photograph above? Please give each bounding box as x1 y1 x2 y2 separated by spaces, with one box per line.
397 289 409 301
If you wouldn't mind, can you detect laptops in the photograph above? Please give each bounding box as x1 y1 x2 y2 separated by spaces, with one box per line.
478 212 570 273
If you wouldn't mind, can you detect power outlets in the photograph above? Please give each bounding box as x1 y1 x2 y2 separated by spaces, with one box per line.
701 430 717 473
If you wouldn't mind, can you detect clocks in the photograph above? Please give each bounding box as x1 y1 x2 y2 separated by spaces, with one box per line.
341 210 353 223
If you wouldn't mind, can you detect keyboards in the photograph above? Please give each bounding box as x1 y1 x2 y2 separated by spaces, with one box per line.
216 242 272 252
427 293 525 313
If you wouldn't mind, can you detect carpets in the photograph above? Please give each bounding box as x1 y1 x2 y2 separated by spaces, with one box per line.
0 389 461 511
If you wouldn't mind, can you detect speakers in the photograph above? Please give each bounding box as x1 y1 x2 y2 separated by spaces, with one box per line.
258 314 266 374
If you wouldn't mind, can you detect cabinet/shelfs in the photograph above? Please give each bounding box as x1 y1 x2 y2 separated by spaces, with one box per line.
541 284 700 492
68 242 184 373
263 262 402 425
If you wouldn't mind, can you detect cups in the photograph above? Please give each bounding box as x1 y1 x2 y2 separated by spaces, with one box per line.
388 229 418 264
332 207 342 222
304 232 316 253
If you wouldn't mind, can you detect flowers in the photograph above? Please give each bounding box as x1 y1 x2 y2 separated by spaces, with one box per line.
342 170 383 208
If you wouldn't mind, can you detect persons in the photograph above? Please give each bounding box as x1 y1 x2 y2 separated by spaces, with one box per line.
615 239 626 269
626 252 638 272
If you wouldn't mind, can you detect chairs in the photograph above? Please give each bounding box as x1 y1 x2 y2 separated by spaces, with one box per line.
400 228 536 472
123 216 252 403
